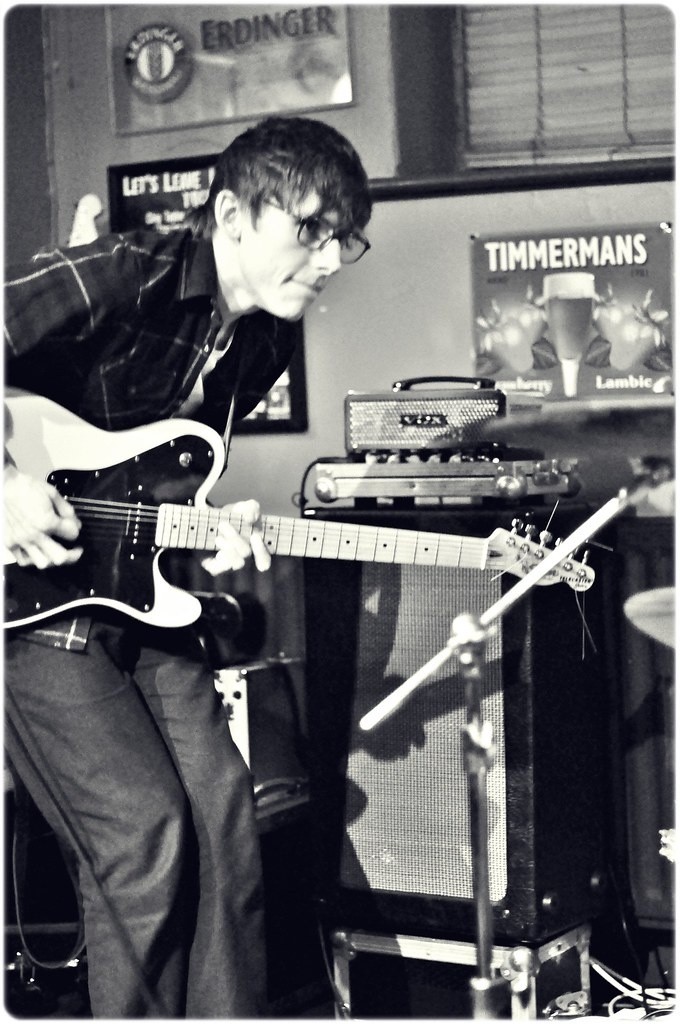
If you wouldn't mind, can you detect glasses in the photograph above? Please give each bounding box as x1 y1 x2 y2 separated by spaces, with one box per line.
255 198 372 264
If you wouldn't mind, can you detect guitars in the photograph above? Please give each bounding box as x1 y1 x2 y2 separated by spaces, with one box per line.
4 381 599 634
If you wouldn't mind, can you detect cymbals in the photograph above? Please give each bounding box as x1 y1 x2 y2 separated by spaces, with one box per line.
623 586 674 650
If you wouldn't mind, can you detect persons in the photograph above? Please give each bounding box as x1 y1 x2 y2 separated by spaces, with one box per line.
4 117 372 1019
